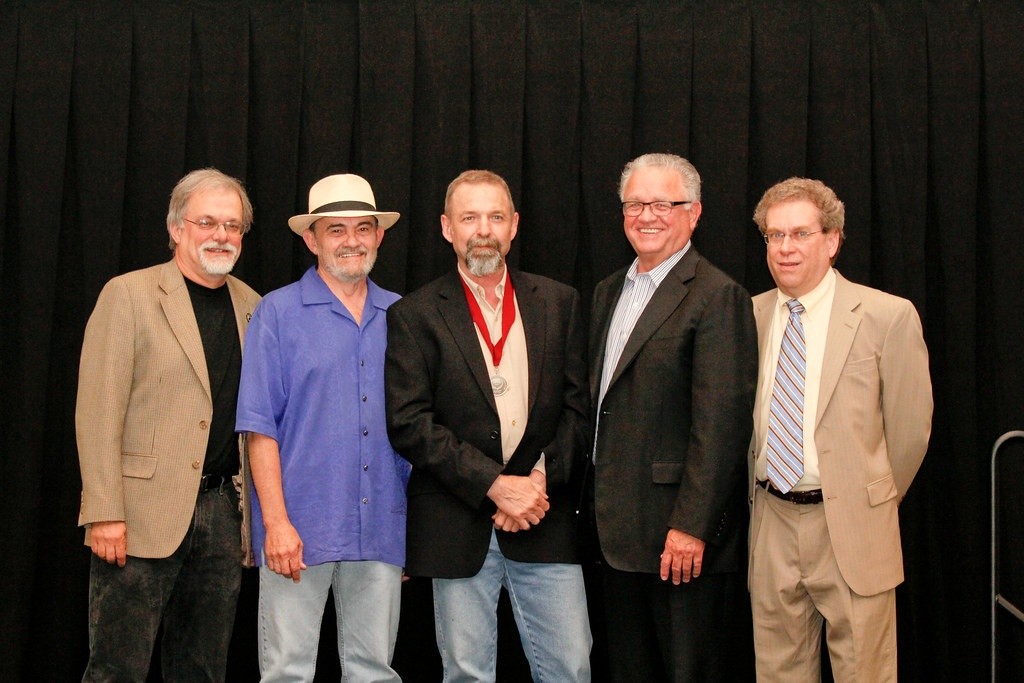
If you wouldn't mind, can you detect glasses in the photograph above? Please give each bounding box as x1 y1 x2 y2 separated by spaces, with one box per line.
621 200 691 217
764 229 821 246
185 216 247 238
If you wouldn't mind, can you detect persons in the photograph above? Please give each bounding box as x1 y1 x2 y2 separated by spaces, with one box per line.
235 174 412 683
750 178 933 683
385 170 593 683
75 170 262 683
585 153 760 683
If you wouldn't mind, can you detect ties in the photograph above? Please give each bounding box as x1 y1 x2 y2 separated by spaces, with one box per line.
766 299 806 494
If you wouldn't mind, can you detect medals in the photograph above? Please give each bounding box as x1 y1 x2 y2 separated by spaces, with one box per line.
490 376 508 397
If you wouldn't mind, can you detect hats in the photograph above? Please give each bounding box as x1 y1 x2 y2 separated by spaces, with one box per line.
288 174 400 237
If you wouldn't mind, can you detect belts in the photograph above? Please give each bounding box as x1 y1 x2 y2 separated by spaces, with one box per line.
759 479 823 504
200 470 234 493
